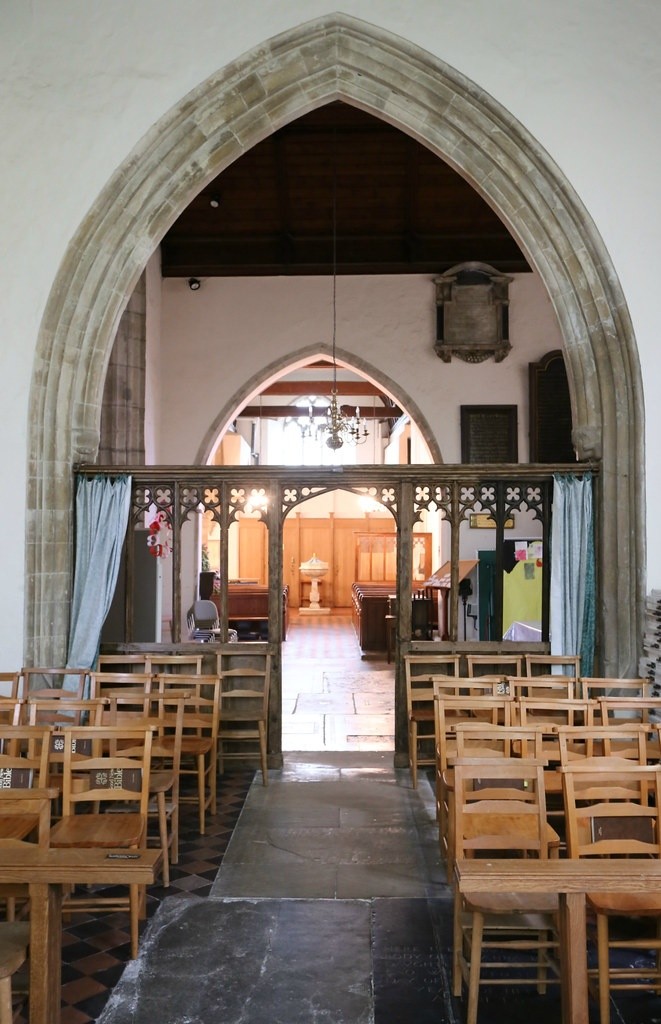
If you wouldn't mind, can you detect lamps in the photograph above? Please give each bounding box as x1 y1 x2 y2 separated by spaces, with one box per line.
280 388 369 452
190 278 200 290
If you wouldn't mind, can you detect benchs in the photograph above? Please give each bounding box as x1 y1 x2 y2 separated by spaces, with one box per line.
209 582 290 640
352 583 439 651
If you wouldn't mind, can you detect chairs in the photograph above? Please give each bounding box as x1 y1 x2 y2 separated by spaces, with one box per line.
0 651 277 1023
186 601 239 643
404 655 661 1024
